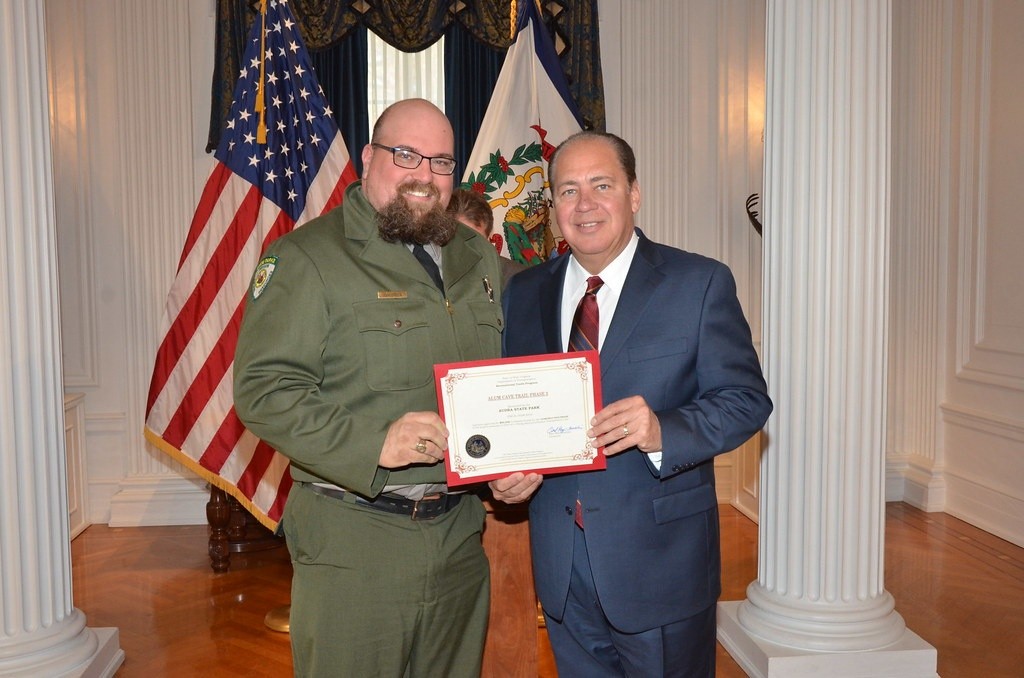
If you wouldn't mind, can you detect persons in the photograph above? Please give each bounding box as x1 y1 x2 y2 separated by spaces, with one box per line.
232 97 505 678
488 130 774 678
445 190 495 241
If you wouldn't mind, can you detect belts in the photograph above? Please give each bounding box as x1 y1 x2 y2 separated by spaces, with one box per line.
303 482 462 517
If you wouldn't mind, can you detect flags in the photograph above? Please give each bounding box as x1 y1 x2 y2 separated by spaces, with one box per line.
143 0 360 535
460 0 588 280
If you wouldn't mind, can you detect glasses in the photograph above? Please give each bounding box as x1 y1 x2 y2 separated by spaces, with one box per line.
371 142 457 175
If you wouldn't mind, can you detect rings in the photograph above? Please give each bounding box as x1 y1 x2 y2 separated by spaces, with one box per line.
623 423 629 437
416 440 427 453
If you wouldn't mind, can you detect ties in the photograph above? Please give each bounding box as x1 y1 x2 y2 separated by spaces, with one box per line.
567 276 604 528
412 243 445 300
